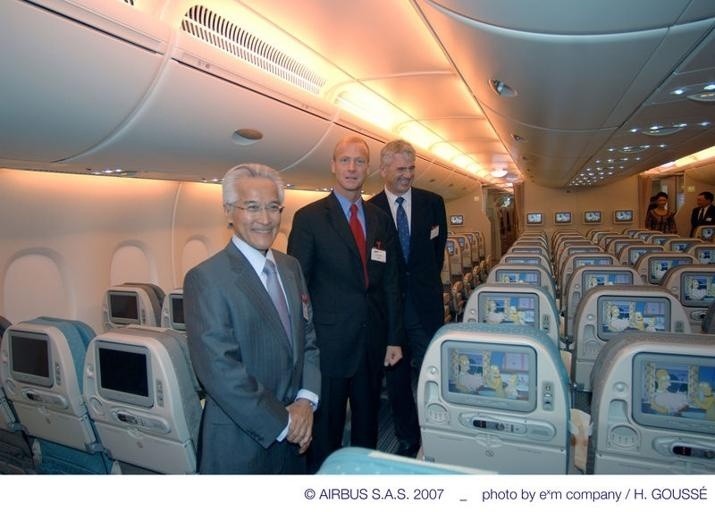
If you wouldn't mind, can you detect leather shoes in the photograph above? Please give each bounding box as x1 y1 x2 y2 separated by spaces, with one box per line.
396 438 420 458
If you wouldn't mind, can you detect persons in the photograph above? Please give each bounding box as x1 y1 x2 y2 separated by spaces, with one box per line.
645 191 678 234
283 135 406 475
367 139 449 458
647 196 657 211
689 191 715 238
184 161 323 474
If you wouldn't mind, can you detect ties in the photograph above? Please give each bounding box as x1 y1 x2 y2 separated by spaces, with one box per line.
396 198 411 265
349 204 369 290
263 258 292 346
699 208 704 223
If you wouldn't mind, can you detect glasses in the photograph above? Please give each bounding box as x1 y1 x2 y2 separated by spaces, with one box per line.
229 203 283 214
